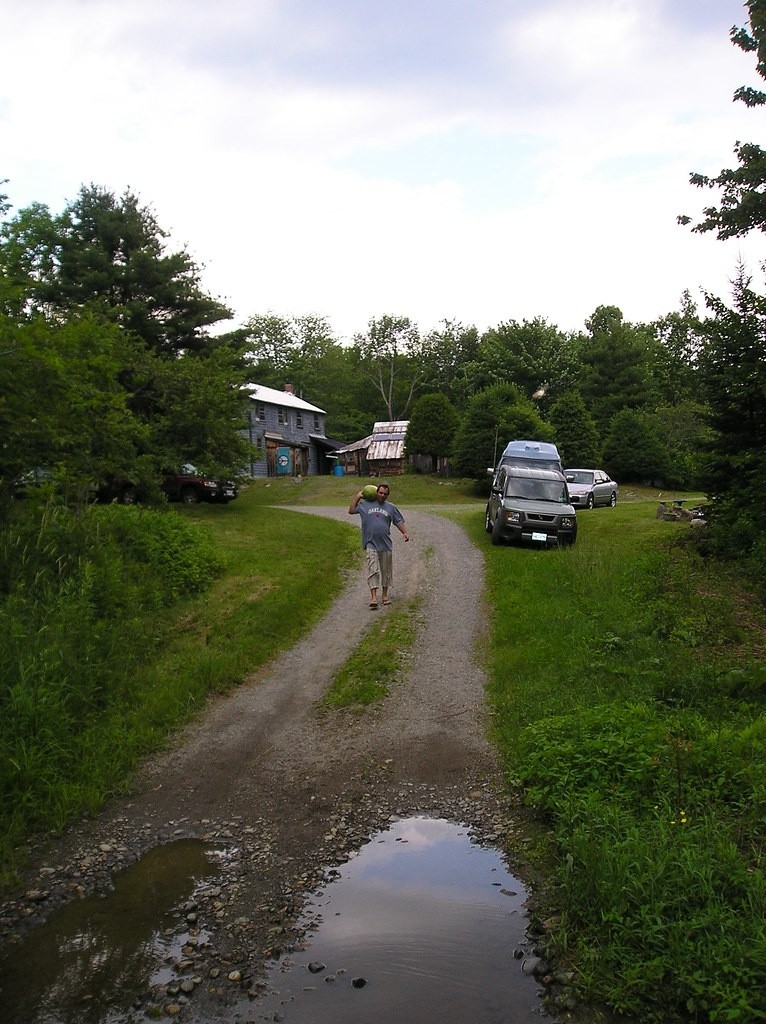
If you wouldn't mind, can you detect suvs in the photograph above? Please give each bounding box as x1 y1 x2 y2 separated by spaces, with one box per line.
486 440 576 487
483 465 581 545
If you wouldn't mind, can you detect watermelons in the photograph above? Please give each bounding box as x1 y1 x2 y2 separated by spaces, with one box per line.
362 485 379 500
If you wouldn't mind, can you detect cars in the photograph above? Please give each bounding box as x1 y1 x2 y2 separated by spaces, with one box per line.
8 462 118 503
111 461 238 506
564 468 619 509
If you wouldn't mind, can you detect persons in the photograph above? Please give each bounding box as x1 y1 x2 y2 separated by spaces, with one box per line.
348 484 409 609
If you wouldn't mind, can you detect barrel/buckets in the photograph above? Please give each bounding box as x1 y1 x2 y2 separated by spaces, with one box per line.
334 466 344 476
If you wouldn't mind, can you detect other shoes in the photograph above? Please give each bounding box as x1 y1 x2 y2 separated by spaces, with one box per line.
382 599 391 605
369 601 379 607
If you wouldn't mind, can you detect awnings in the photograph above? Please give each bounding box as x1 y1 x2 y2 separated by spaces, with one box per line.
309 434 347 451
